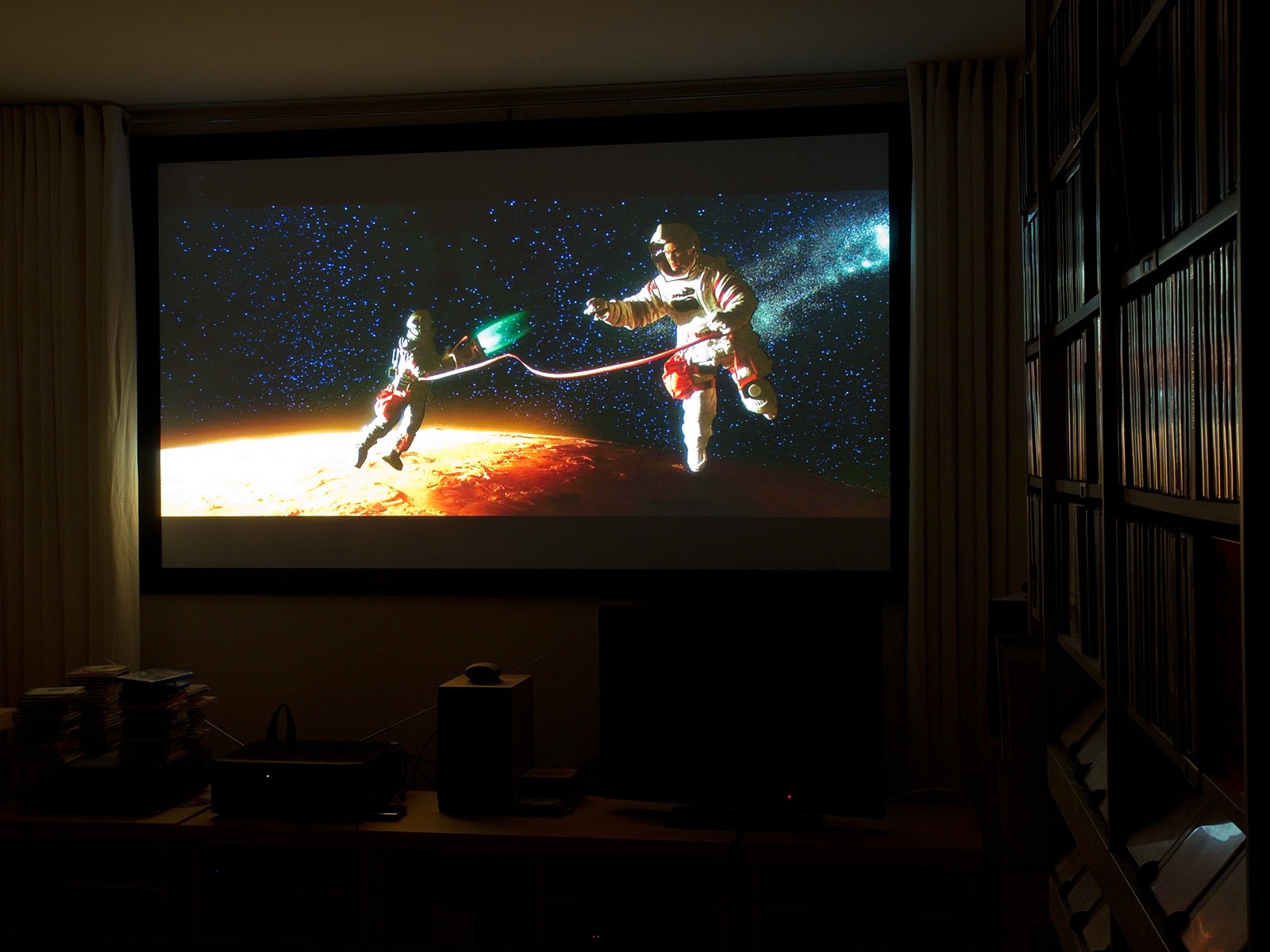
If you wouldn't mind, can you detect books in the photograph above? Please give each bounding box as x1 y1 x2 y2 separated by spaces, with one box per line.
12 664 217 813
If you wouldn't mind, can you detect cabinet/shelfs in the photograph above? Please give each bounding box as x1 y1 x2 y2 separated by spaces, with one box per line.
1 786 986 952
1017 0 1270 952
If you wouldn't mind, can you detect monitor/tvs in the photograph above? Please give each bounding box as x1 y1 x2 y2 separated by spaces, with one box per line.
597 602 886 819
131 108 910 598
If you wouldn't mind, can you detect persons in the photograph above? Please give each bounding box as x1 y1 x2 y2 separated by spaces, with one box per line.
354 311 478 471
583 223 777 473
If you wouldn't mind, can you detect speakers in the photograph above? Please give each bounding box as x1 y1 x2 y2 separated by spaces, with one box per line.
436 676 535 820
